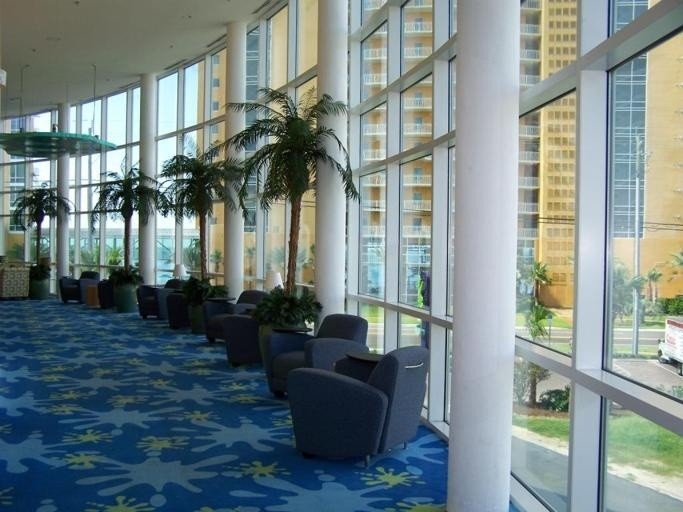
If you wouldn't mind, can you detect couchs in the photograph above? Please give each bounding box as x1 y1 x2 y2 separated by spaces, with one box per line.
136 279 277 369
261 313 429 469
59 270 142 310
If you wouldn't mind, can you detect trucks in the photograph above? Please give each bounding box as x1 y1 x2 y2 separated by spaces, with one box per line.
657 315 683 376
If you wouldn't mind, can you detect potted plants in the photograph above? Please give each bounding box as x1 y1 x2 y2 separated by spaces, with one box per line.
155 133 248 334
88 155 168 312
9 182 77 299
221 87 361 378
